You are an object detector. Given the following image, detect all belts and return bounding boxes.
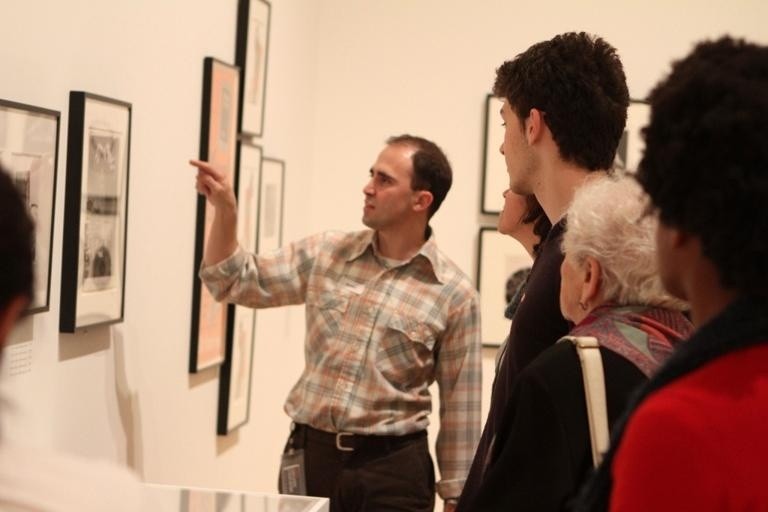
[294,422,394,454]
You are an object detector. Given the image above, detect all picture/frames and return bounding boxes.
[480,93,652,216]
[476,226,534,348]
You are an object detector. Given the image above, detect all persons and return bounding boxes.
[187,136,483,510]
[1,164,144,487]
[490,33,633,479]
[455,169,697,512]
[610,33,768,511]
[496,178,552,333]
